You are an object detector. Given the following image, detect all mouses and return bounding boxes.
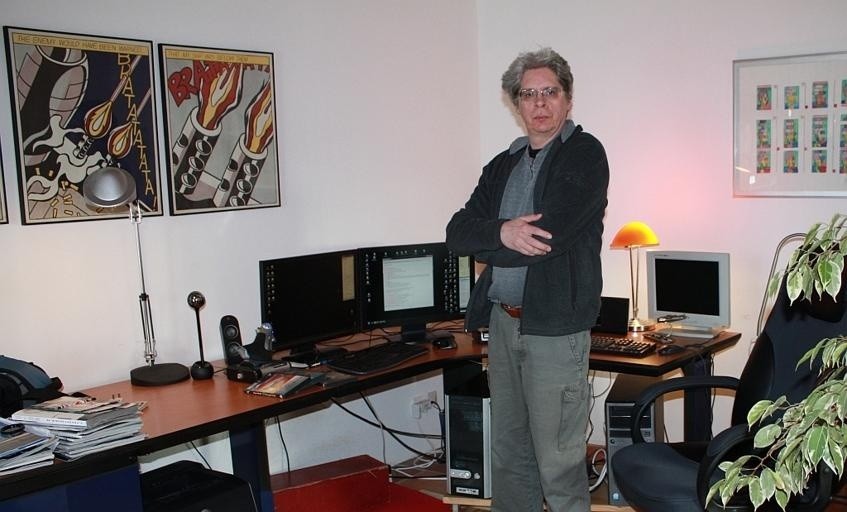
[658,344,682,356]
[428,329,457,350]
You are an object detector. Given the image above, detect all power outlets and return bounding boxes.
[412,394,429,418]
[425,390,439,412]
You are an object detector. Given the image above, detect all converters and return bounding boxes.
[410,401,420,419]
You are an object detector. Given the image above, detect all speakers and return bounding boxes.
[221,315,244,366]
[591,296,630,335]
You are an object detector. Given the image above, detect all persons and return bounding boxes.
[445,46,611,512]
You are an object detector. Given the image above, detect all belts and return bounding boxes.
[498,303,526,321]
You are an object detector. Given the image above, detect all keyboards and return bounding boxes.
[591,336,656,358]
[329,341,429,375]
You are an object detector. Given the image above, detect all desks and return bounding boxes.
[209,322,742,512]
[1,366,332,512]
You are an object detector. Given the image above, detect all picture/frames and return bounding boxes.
[155,43,282,216]
[732,50,847,199]
[4,27,164,227]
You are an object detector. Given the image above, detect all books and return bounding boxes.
[244,369,356,398]
[11,395,150,462]
[0,416,61,477]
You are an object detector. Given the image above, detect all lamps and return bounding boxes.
[608,224,660,332]
[83,167,189,387]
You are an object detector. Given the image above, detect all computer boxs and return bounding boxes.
[606,375,664,506]
[442,381,490,498]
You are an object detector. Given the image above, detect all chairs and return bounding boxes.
[611,242,846,511]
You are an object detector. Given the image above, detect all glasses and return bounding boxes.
[519,86,567,103]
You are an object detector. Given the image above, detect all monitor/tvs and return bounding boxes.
[645,251,731,339]
[358,242,460,344]
[258,249,358,369]
